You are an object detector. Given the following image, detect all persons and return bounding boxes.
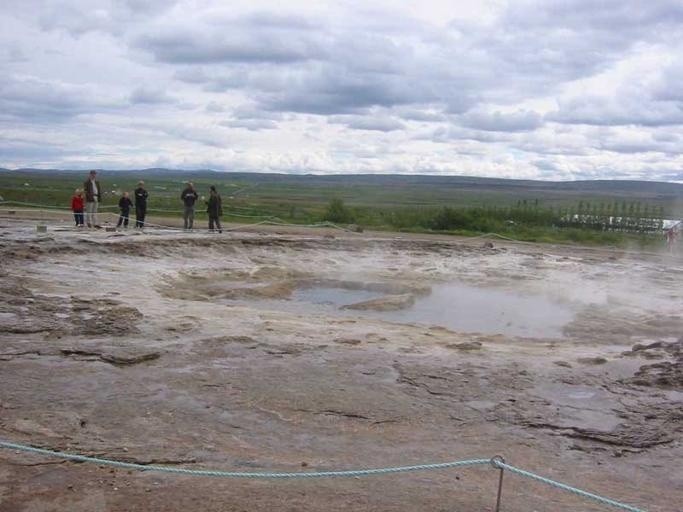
[115,191,133,230]
[132,180,148,230]
[179,182,198,232]
[82,170,100,228]
[200,185,222,233]
[69,188,85,227]
